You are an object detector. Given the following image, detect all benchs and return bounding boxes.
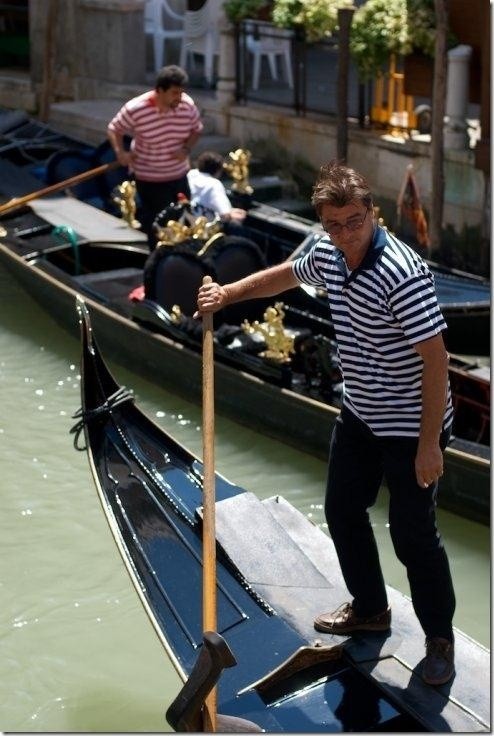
[46,135,144,220]
[144,235,312,374]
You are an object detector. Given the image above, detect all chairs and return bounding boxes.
[179,0,220,83]
[245,19,294,91]
[144,0,195,74]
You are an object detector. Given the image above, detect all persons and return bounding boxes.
[187,152,249,224]
[191,160,460,689]
[105,63,206,250]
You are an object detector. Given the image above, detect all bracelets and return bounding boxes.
[182,146,191,153]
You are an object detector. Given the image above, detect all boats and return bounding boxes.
[69,293,492,733]
[1,106,491,525]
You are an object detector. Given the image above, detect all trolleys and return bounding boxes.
[371,47,432,134]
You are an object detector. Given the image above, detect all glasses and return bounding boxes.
[321,209,367,235]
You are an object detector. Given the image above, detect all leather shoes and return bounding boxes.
[314,601,391,633]
[423,631,454,685]
